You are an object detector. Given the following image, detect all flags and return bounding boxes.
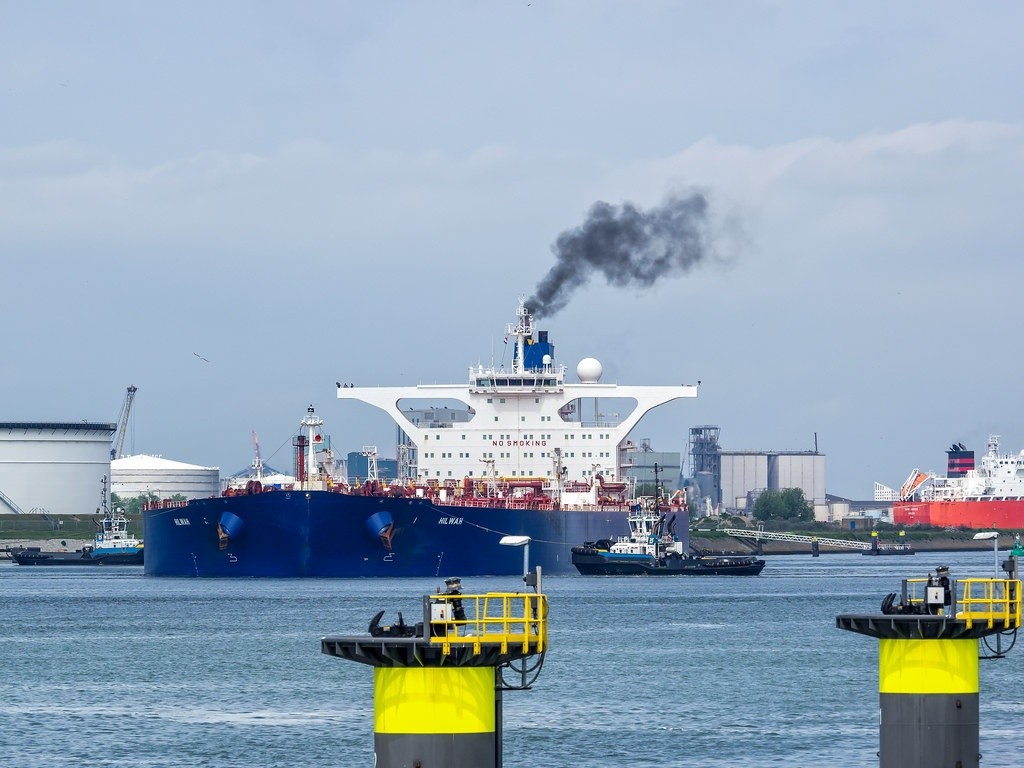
[504,335,508,344]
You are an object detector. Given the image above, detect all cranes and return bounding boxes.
[110,383,139,460]
[723,525,912,553]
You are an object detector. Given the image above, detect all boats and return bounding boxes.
[140,291,703,582]
[892,434,1024,533]
[569,458,767,578]
[1009,532,1024,559]
[5,473,143,567]
[596,473,626,493]
